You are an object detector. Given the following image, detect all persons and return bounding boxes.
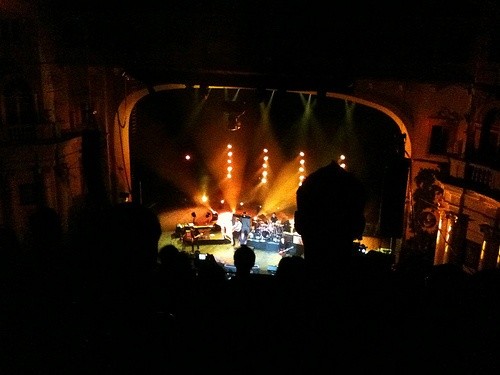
[393,249,427,279]
[20,202,63,252]
[267,257,312,299]
[254,161,418,375]
[1,194,231,374]
[157,206,305,281]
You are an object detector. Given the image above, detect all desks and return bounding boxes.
[247,238,285,252]
